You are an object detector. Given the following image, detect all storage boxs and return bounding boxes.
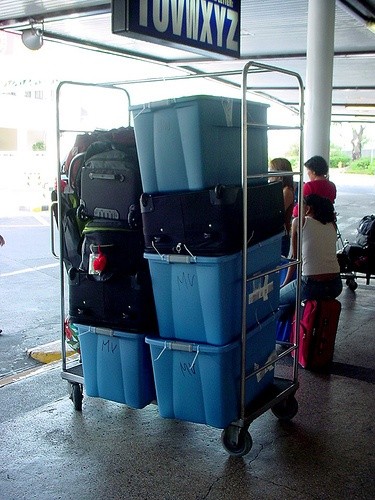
[144,307,285,428]
[143,229,287,345]
[72,323,154,409]
[128,94,270,193]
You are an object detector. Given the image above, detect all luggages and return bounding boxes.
[67,267,158,335]
[76,140,143,230]
[290,299,341,369]
[140,180,285,257]
[78,217,150,273]
[339,244,374,275]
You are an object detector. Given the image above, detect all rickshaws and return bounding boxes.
[52,60,305,458]
[335,224,375,290]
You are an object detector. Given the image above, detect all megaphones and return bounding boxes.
[21,28,44,50]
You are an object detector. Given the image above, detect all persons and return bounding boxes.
[279,194,342,307]
[270,158,294,257]
[292,155,336,217]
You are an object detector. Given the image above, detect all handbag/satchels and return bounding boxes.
[357,215,375,234]
[356,235,375,246]
[61,126,134,195]
[50,178,84,273]
